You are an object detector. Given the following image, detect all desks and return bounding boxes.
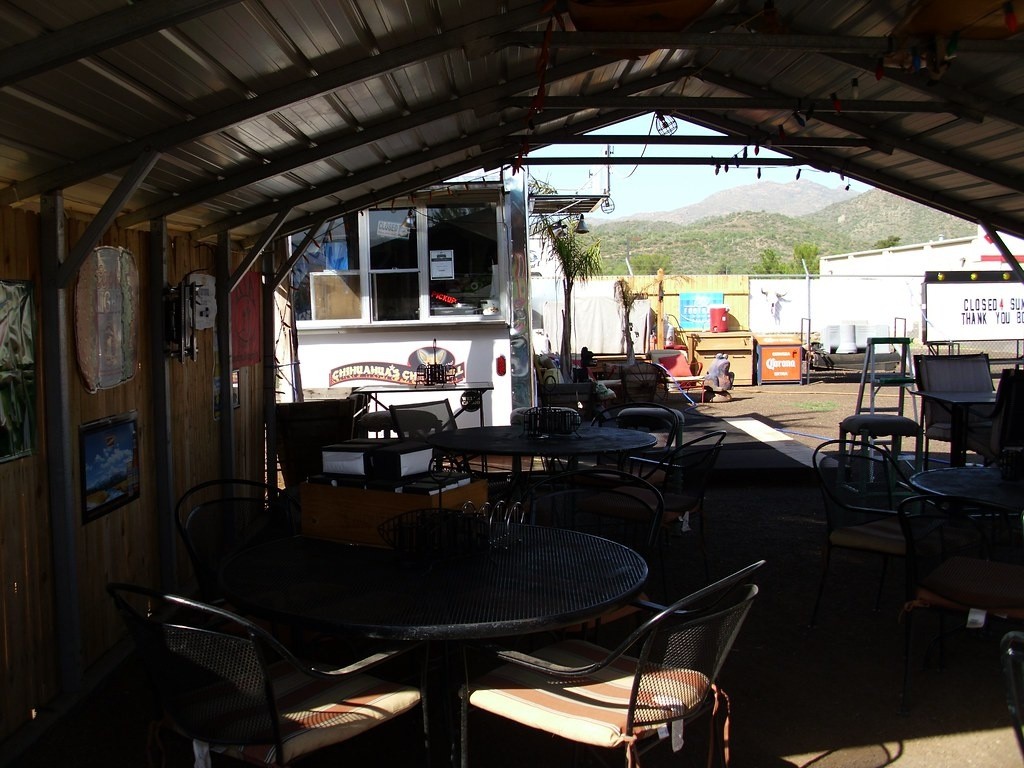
[351,381,494,475]
[217,519,649,768]
[909,464,1024,531]
[908,391,996,465]
[426,425,659,500]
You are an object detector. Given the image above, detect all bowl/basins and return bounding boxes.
[481,299,499,315]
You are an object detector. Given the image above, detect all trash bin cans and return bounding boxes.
[688,332,753,387]
[757,344,803,384]
[277,394,366,487]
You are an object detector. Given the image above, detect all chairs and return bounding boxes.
[177,478,361,672]
[646,349,705,403]
[456,559,766,768]
[811,351,1024,757]
[105,580,434,767]
[387,401,728,647]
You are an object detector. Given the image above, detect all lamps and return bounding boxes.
[575,213,589,234]
[164,281,199,362]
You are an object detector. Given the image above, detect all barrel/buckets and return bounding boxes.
[709,304,729,332]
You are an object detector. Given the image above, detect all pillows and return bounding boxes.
[659,354,693,377]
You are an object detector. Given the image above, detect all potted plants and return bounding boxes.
[525,179,607,416]
[602,274,695,406]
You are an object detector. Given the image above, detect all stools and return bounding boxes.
[839,417,923,512]
